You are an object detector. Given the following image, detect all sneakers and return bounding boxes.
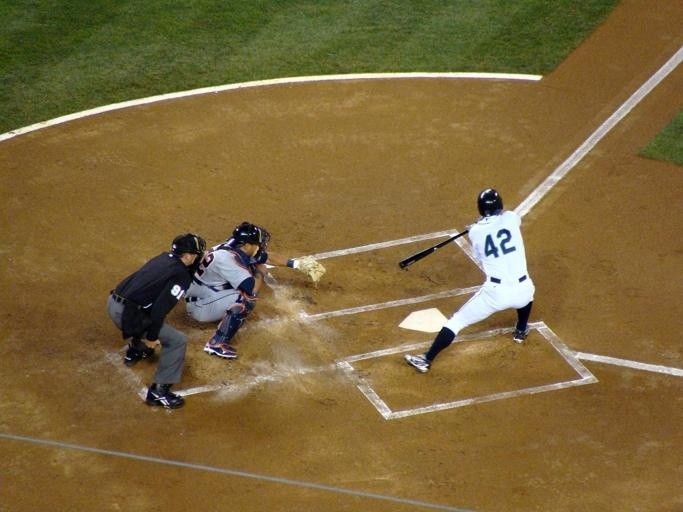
[122,343,157,367]
[146,387,186,409]
[404,353,432,374]
[513,324,530,344]
[204,337,239,361]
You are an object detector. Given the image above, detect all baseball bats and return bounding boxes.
[399,229,469,268]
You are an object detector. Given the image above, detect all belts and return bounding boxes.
[491,275,527,284]
[183,297,198,302]
[108,290,153,312]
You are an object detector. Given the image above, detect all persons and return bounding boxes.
[404,188,535,373]
[107,234,208,409]
[185,222,304,359]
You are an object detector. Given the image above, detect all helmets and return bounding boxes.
[171,233,206,255]
[232,221,270,247]
[476,187,504,218]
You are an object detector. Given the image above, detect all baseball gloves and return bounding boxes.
[293,257,326,281]
